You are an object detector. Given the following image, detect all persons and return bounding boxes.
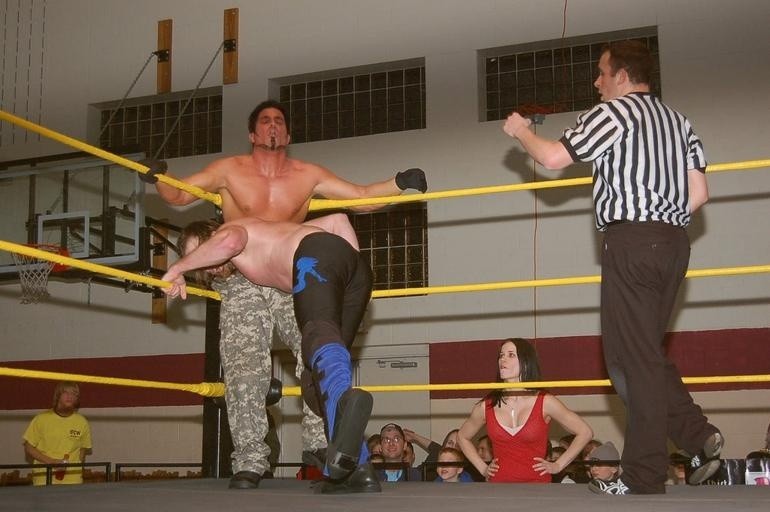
[503,43,724,493]
[457,338,594,483]
[366,423,620,483]
[159,213,381,495]
[24,383,92,485]
[664,449,692,486]
[131,100,428,489]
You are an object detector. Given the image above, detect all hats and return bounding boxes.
[589,442,619,461]
[381,424,403,437]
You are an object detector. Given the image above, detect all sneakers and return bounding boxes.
[589,476,637,496]
[686,433,723,485]
[228,471,260,489]
[310,389,381,480]
[314,463,382,494]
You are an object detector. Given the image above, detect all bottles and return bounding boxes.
[55,453,69,480]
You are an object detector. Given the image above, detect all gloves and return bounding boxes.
[395,168,427,194]
[129,159,167,183]
